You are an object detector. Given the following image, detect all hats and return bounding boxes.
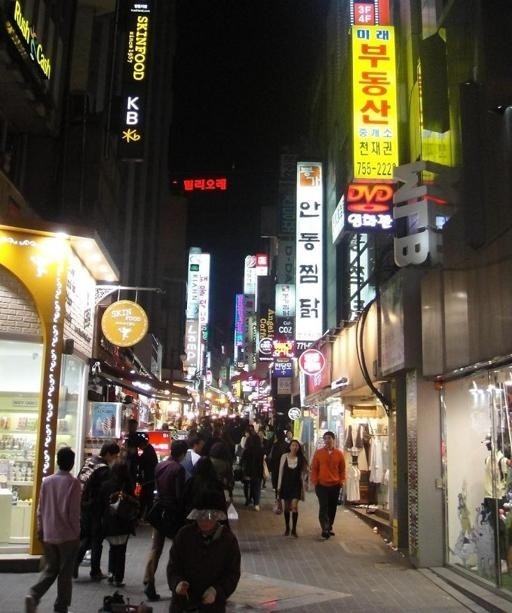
[186,488,228,521]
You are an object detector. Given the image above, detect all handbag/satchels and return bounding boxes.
[147,501,177,538]
[109,479,141,521]
[272,489,283,514]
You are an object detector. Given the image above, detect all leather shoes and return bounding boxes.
[321,531,329,537]
[329,529,334,535]
[144,582,160,600]
[92,571,107,578]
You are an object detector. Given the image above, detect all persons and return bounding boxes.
[166,486,242,611]
[23,446,83,612]
[142,436,235,602]
[78,444,140,586]
[187,412,293,510]
[310,431,348,540]
[275,438,309,539]
[153,412,189,430]
[481,432,509,575]
[119,418,159,522]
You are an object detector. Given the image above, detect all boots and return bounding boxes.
[284,511,289,535]
[291,511,298,537]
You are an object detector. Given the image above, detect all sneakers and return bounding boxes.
[24,594,37,612]
[254,504,260,511]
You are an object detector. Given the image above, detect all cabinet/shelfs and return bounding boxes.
[0,391,78,544]
